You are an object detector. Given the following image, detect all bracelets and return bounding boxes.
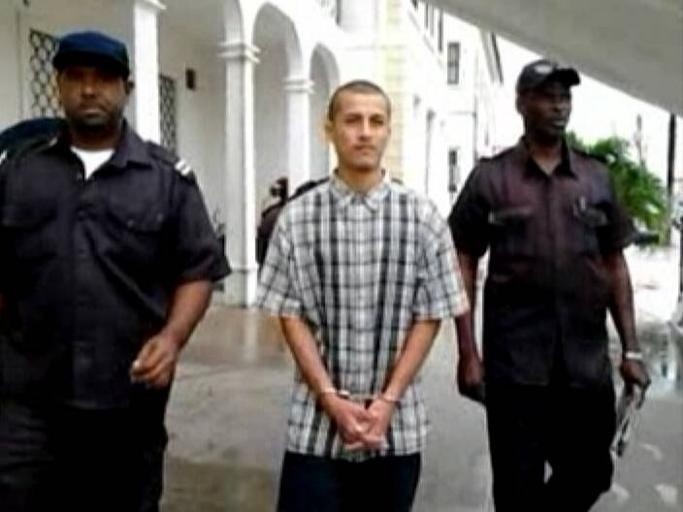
[621,350,643,362]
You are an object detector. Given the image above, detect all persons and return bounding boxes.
[444,58,654,511]
[0,30,233,511]
[247,78,473,512]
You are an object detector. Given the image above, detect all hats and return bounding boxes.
[51,31,130,81]
[517,59,580,94]
